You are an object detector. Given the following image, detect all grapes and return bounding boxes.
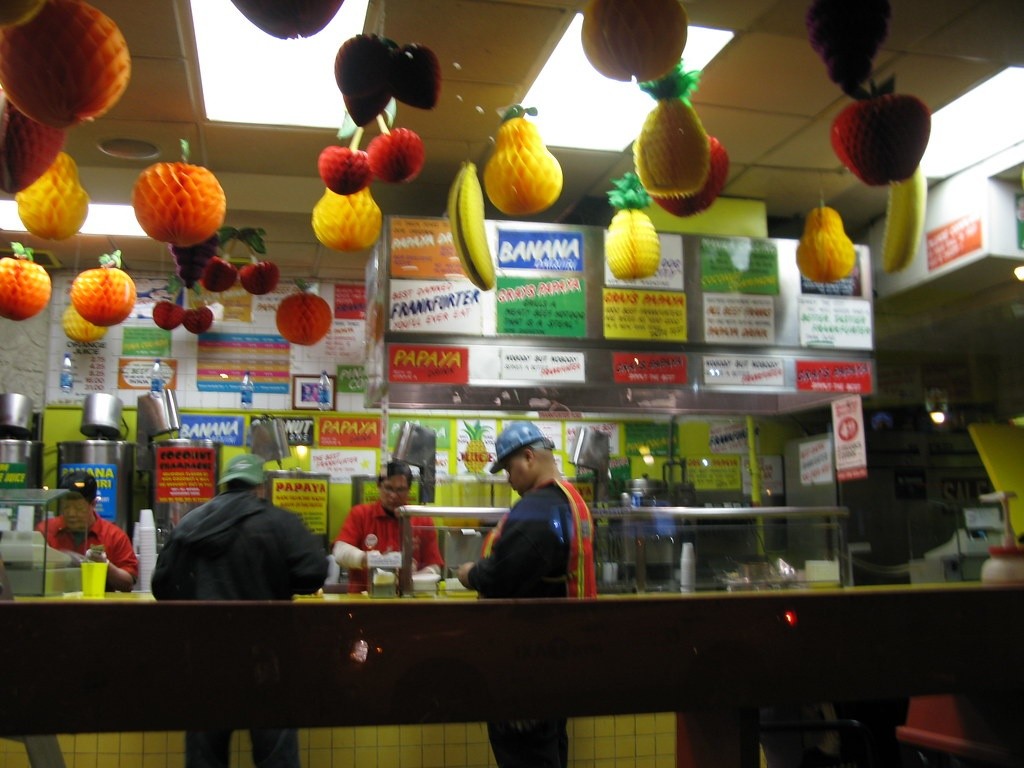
[806,0,891,92]
[169,232,219,290]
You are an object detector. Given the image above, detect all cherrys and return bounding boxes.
[318,110,424,194]
[151,278,213,334]
[198,233,279,295]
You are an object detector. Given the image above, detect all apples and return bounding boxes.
[828,78,931,183]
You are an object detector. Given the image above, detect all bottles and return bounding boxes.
[318,370,332,411]
[981,545,1024,584]
[60,352,74,403]
[150,359,162,392]
[241,372,254,409]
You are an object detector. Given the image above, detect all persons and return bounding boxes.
[150,453,329,767]
[459,421,596,768]
[37,471,138,594]
[331,462,446,594]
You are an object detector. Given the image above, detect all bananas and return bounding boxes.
[448,161,495,292]
[883,169,926,271]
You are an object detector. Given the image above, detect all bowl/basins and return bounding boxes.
[412,573,441,598]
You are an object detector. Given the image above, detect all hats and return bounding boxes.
[217,455,268,485]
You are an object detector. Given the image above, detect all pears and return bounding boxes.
[483,109,562,215]
[796,191,855,283]
[312,181,382,253]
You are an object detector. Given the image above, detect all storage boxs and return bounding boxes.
[0,489,88,596]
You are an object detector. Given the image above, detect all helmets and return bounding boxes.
[489,421,546,475]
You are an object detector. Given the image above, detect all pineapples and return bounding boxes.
[632,55,712,198]
[604,171,661,279]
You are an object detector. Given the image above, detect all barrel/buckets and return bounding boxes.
[0,389,331,550]
[395,421,437,469]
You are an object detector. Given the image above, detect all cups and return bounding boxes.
[680,542,695,593]
[132,509,158,593]
[601,561,618,583]
[81,562,108,599]
